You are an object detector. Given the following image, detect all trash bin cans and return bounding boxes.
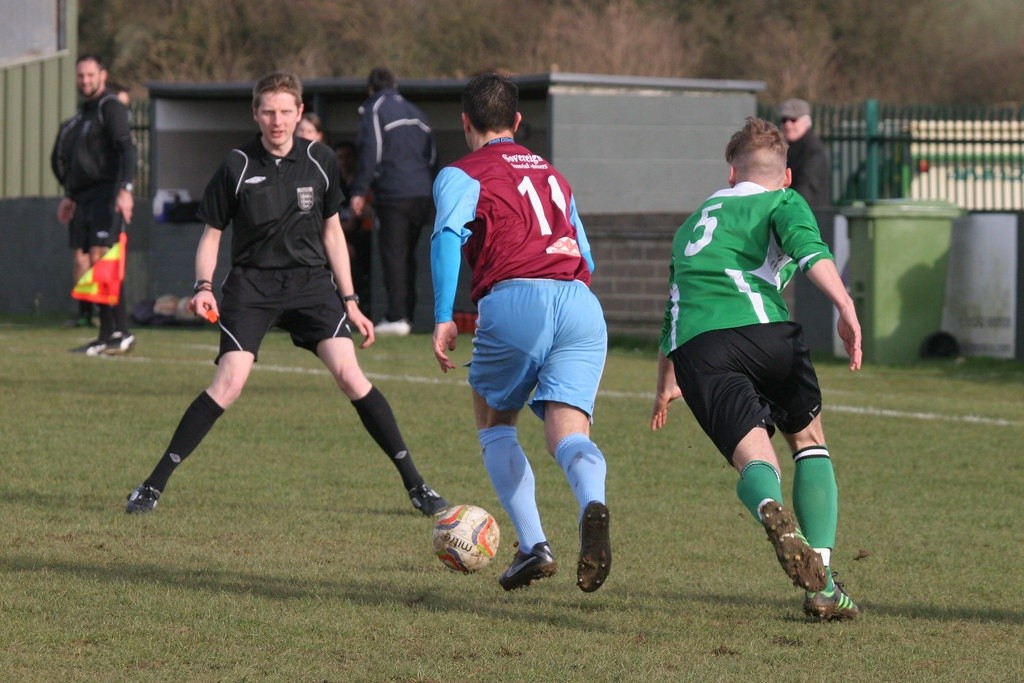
[840,198,960,367]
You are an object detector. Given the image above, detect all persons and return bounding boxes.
[51,55,139,358]
[432,72,613,592]
[122,65,448,517]
[652,99,862,621]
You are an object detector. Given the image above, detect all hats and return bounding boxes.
[775,98,811,121]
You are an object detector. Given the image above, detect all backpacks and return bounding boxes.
[51,110,109,195]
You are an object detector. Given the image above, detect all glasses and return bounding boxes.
[780,118,797,123]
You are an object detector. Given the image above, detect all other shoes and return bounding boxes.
[73,332,138,356]
[372,318,412,335]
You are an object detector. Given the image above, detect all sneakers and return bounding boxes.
[758,500,827,593]
[408,480,448,518]
[123,484,162,515]
[804,572,859,619]
[499,541,558,591]
[577,500,612,593]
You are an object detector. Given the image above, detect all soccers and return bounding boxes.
[431,504,502,573]
[153,293,199,320]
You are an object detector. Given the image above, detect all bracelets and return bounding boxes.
[195,287,213,292]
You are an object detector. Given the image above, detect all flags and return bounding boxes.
[69,232,128,305]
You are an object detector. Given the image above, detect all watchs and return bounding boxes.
[342,294,360,305]
[123,182,133,192]
[194,280,213,289]
